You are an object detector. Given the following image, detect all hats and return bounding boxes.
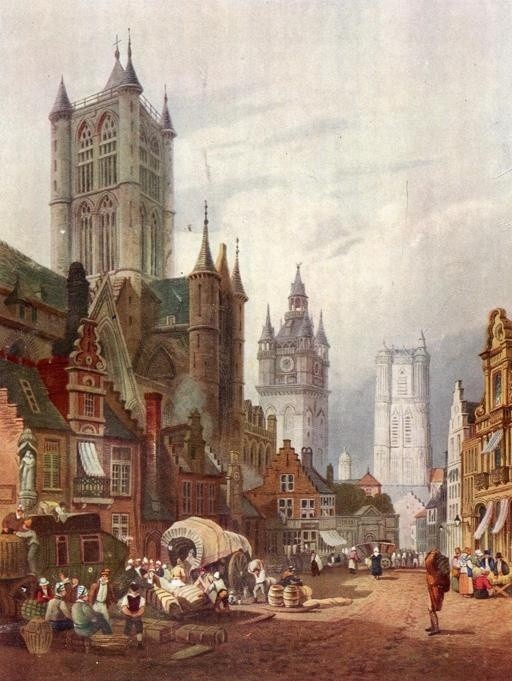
[55,582,64,593]
[483,550,489,554]
[100,568,111,575]
[76,585,88,599]
[495,553,504,558]
[252,568,259,572]
[39,577,49,586]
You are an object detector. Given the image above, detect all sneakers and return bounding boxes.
[425,627,440,636]
